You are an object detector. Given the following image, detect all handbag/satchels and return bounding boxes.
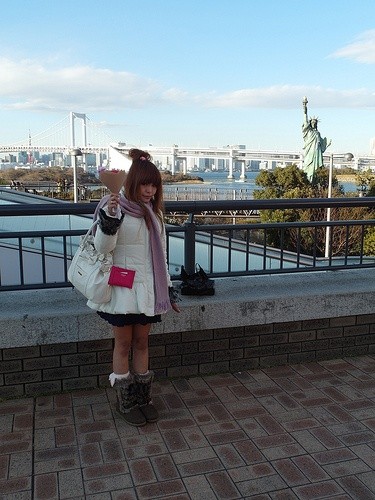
[66,218,112,304]
[180,263,216,296]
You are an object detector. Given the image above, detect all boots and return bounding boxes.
[133,370,159,423]
[110,373,147,427]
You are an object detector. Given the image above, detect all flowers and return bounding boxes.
[95,165,127,216]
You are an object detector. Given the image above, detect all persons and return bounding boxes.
[11,180,24,186]
[86,148,183,427]
[57,179,87,200]
[300,96,331,183]
